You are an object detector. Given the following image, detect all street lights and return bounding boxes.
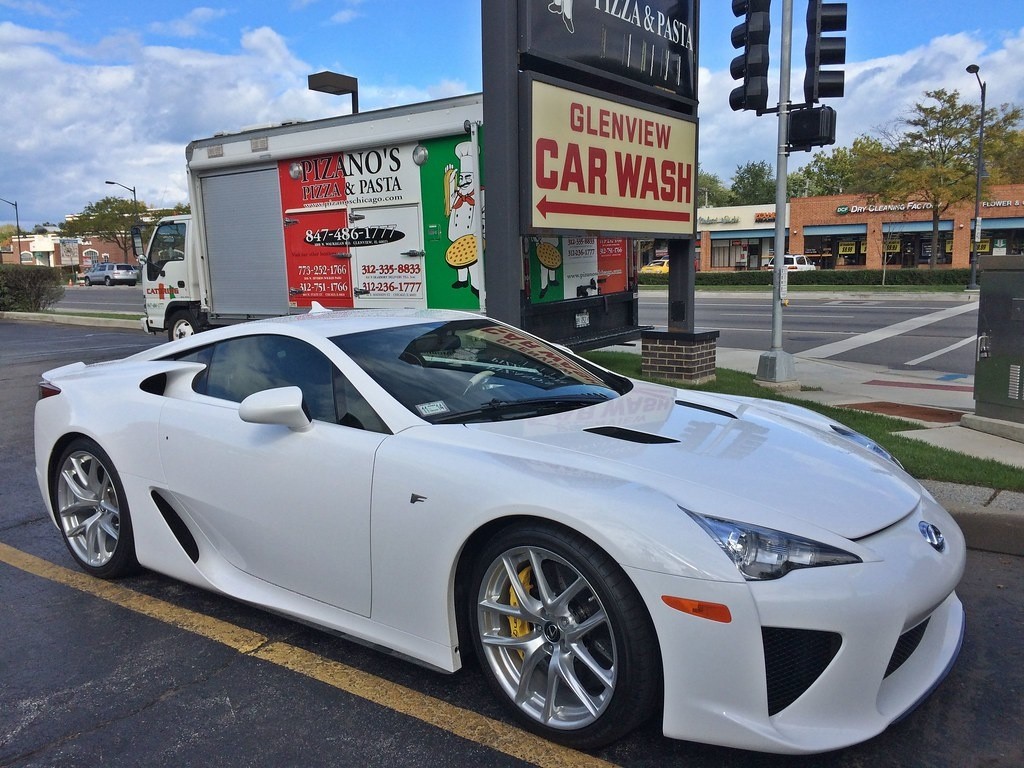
[107,179,140,226]
[963,63,989,290]
[0,198,22,264]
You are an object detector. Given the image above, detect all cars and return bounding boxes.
[84,262,138,286]
[767,254,816,271]
[769,254,769,255]
[641,255,669,274]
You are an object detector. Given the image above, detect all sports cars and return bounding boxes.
[33,303,969,759]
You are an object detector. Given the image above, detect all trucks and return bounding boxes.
[139,89,657,357]
